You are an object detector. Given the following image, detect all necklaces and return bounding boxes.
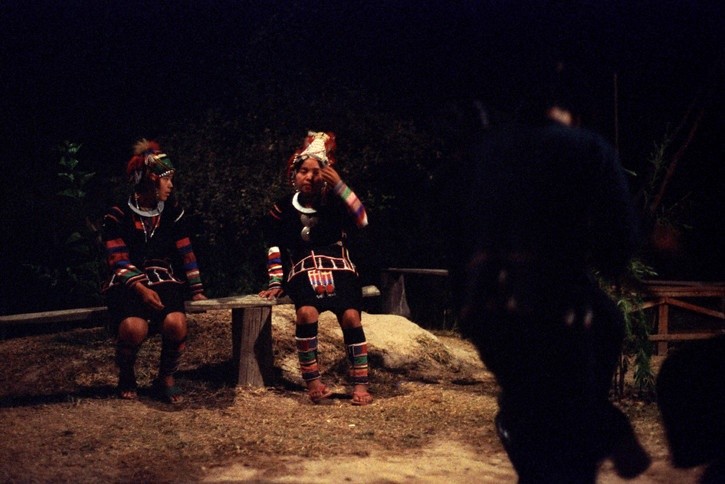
[293,191,330,243]
[126,192,166,217]
[135,192,163,246]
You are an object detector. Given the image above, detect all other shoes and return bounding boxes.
[118,381,139,399]
[161,384,184,403]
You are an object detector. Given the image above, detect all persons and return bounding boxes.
[433,68,659,484]
[258,130,378,407]
[100,140,209,406]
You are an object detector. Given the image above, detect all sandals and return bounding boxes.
[308,384,332,403]
[350,391,374,405]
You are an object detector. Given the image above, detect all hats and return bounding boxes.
[286,129,338,199]
[126,137,176,203]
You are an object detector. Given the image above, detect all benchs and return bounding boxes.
[132,280,382,389]
